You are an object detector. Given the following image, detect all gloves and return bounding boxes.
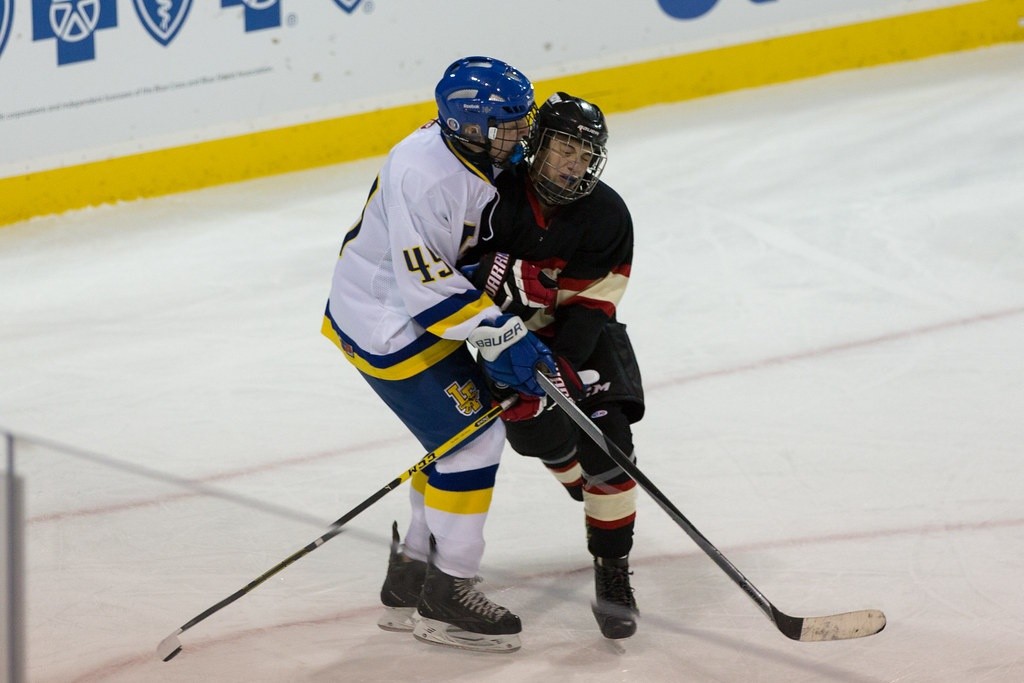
[490,353,585,422]
[466,314,557,397]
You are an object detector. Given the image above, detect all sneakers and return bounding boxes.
[377,521,429,629]
[412,534,523,653]
[592,555,639,652]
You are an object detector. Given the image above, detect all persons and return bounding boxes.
[321,55,559,653]
[477,92,644,652]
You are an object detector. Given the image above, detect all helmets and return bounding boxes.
[528,92,607,206]
[434,56,538,168]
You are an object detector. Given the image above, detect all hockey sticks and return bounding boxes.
[155,394,520,665]
[536,368,886,643]
